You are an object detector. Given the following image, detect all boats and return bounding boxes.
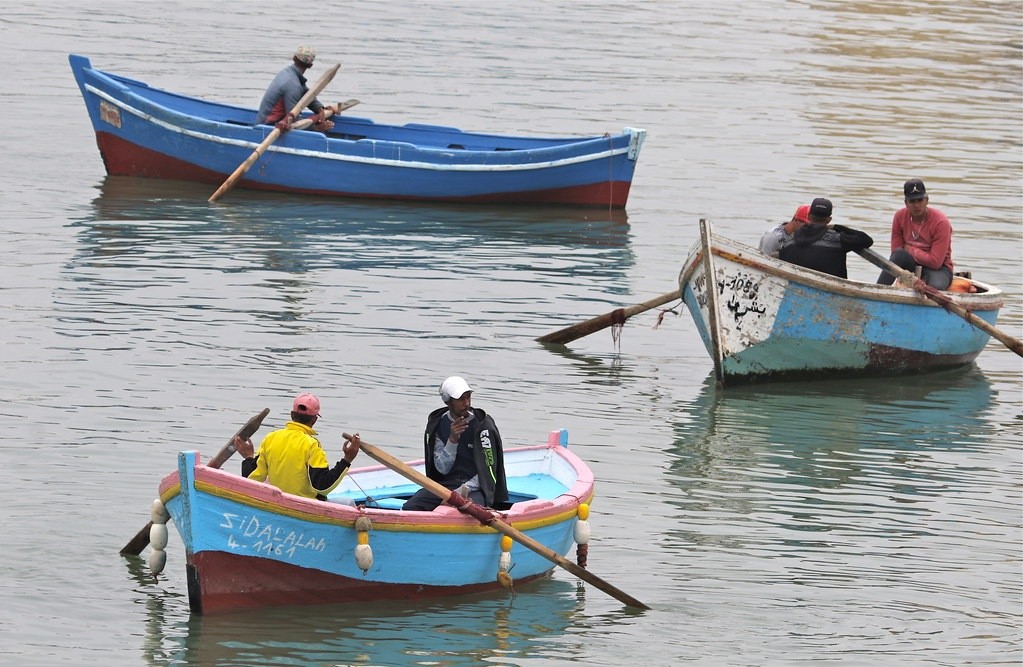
[161,429,598,621]
[674,217,1005,388]
[67,54,644,216]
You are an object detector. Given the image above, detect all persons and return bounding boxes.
[781,198,873,279]
[759,204,812,258]
[401,376,507,510]
[255,46,334,132]
[877,179,953,289]
[233,393,360,501]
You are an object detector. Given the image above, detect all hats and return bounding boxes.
[293,392,322,419]
[295,47,316,64]
[441,376,475,400]
[810,198,833,217]
[904,178,926,199]
[794,205,811,224]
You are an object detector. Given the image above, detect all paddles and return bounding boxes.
[534,290,679,344]
[119,408,270,554]
[338,430,649,609]
[208,62,360,202]
[854,247,1023,358]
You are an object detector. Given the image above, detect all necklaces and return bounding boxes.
[910,216,925,240]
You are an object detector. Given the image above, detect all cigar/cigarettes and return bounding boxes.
[355,435,360,439]
[467,410,473,415]
